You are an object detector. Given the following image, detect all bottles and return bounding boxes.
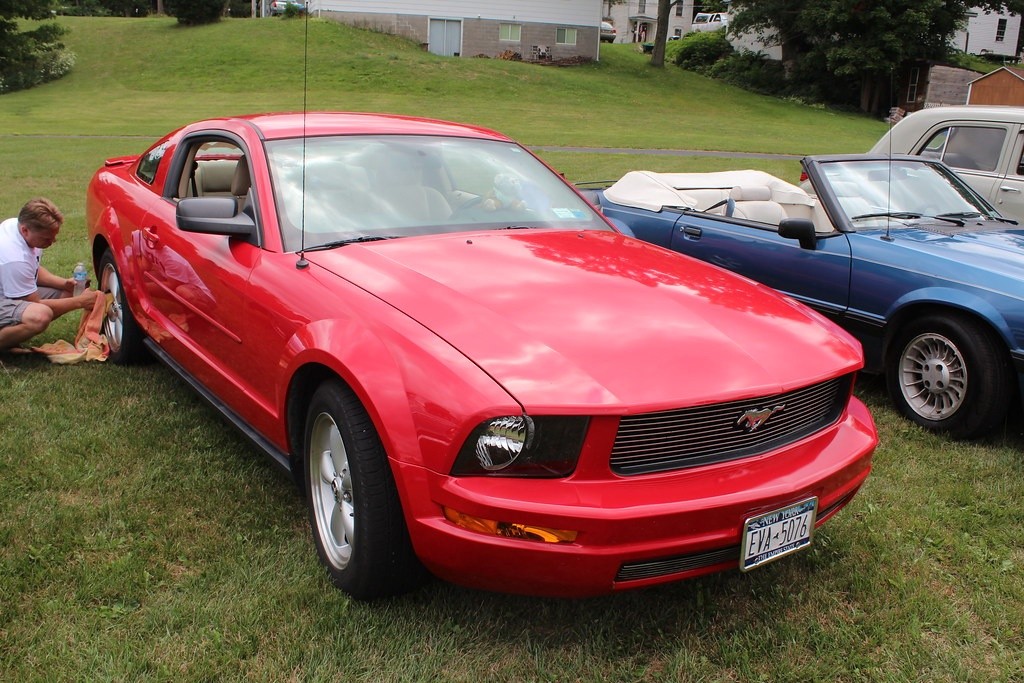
[72,262,88,297]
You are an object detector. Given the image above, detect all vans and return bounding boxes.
[691,12,728,34]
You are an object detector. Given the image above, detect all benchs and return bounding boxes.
[188,159,240,197]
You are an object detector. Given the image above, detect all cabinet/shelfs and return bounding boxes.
[532,45,552,62]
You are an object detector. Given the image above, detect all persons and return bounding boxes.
[631,25,647,44]
[0,197,112,354]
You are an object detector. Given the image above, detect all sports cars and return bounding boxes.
[571,152,1024,440]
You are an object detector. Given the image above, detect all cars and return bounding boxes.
[800,105,1024,231]
[85,111,878,603]
[600,22,617,43]
[269,0,302,17]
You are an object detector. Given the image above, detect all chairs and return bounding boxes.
[231,154,251,216]
[722,184,789,225]
[811,182,878,233]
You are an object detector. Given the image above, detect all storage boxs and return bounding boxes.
[889,107,905,122]
